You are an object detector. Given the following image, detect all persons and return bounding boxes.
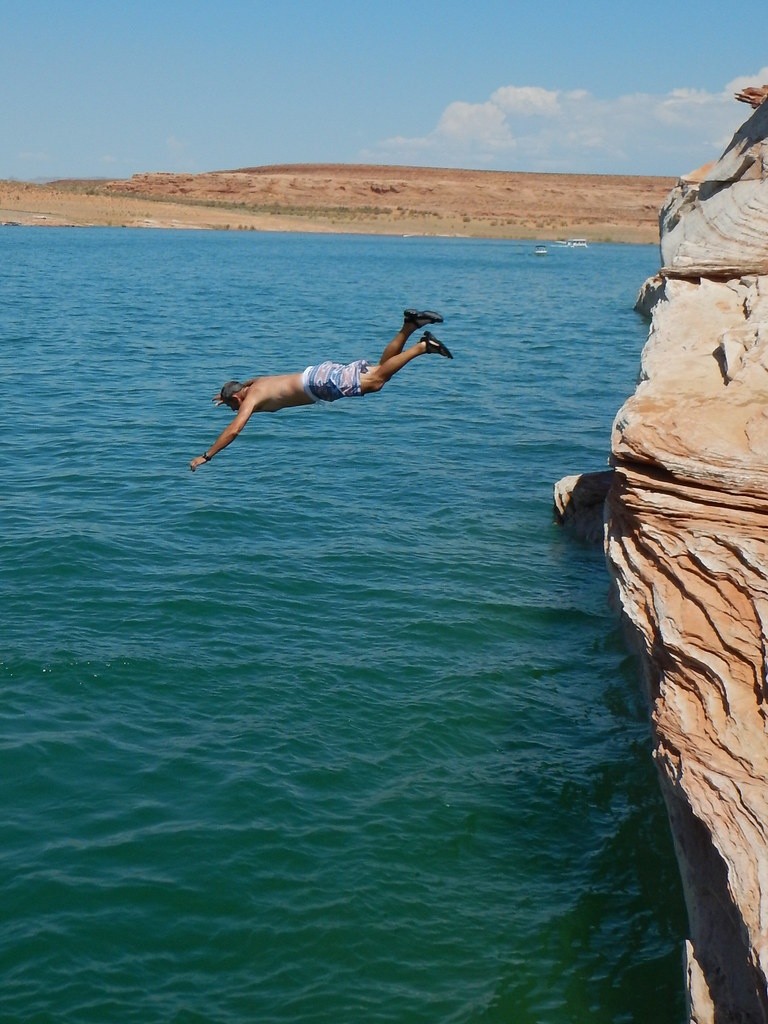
[190,309,454,473]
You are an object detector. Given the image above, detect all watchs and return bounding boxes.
[202,451,211,462]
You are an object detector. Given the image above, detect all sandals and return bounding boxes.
[404,309,444,328]
[420,331,454,359]
